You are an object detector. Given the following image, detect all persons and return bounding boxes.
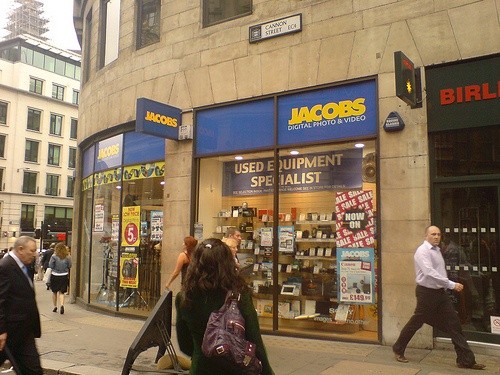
[392,225,486,370]
[0,235,72,314]
[438,232,491,332]
[0,236,44,375]
[165,227,276,375]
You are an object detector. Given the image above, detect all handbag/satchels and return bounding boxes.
[43,268,52,283]
[201,288,262,375]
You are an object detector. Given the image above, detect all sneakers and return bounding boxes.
[395,352,408,363]
[458,363,484,369]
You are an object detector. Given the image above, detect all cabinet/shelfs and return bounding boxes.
[294,220,336,260]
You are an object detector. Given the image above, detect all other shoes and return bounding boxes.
[53,307,57,312]
[61,306,64,314]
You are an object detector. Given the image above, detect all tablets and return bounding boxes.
[281,285,295,294]
